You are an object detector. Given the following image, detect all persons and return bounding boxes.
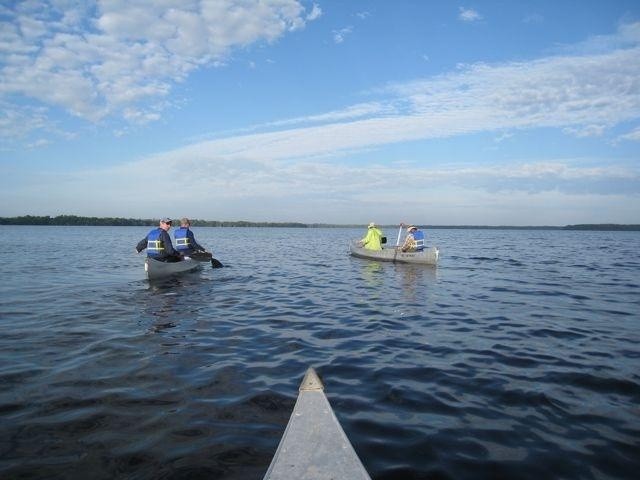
[134,216,191,263]
[355,222,383,250]
[401,224,424,252]
[173,217,207,255]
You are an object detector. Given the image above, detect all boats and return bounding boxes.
[348,239,440,265]
[143,252,203,278]
[261,364,372,480]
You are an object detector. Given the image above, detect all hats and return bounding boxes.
[180,217,190,224]
[407,224,417,233]
[160,217,173,223]
[368,223,377,228]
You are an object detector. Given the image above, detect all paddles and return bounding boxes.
[141,249,212,263]
[202,250,222,266]
